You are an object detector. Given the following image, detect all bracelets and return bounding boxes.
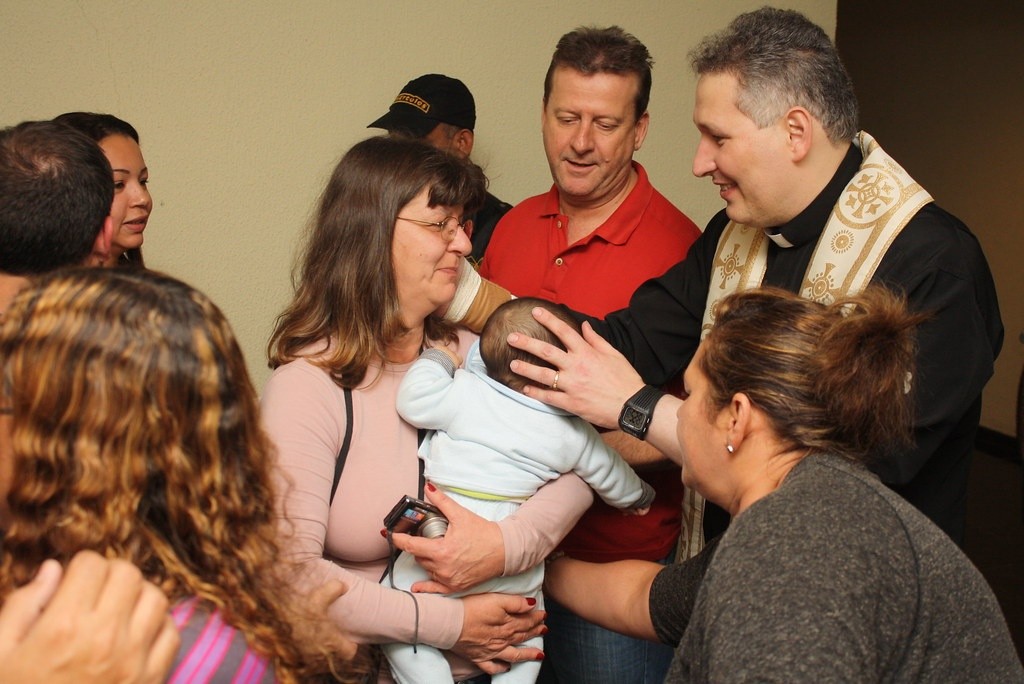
[542,550,564,594]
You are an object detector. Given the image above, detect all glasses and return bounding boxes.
[395,213,474,243]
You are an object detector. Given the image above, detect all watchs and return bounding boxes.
[618,385,668,441]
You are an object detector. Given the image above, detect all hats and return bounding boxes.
[367,73,475,139]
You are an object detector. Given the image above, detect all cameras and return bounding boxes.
[384,495,449,539]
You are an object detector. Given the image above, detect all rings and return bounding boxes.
[553,371,560,391]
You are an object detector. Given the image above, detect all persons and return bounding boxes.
[542,286,1024,684]
[257,136,596,684]
[379,296,656,684]
[477,25,703,684]
[366,73,515,270]
[0,111,375,684]
[428,7,1003,567]
